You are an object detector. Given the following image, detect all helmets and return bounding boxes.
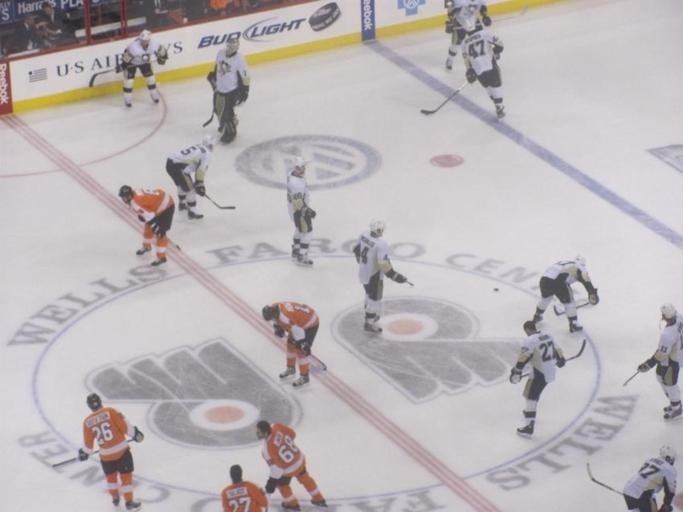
[202,135,216,152]
[660,445,675,465]
[87,394,101,409]
[225,39,240,55]
[140,29,151,41]
[370,219,385,237]
[263,305,276,320]
[661,303,676,319]
[119,185,133,204]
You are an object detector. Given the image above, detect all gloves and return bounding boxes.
[79,449,88,461]
[265,479,276,493]
[639,363,650,373]
[196,186,205,196]
[275,329,284,337]
[510,368,522,384]
[158,58,166,64]
[133,427,144,442]
[395,274,407,283]
[306,208,315,218]
[445,22,454,33]
[466,68,476,83]
[588,289,598,305]
[556,358,565,368]
[116,61,128,72]
[296,339,310,356]
[482,16,491,26]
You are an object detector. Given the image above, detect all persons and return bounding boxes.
[207,37,250,143]
[460,19,505,118]
[445,0,492,70]
[263,301,319,387]
[353,226,407,332]
[222,465,268,512]
[119,185,175,266]
[256,420,325,509]
[166,135,216,219]
[623,448,678,512]
[509,321,566,434]
[7,0,258,54]
[78,394,144,510]
[116,30,168,107]
[637,305,683,419]
[532,254,599,333]
[286,157,316,264]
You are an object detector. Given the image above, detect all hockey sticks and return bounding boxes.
[586,464,623,499]
[422,80,467,114]
[553,303,590,316]
[520,341,586,377]
[203,98,216,127]
[280,332,327,373]
[89,70,117,88]
[204,194,234,209]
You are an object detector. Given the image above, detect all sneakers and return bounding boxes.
[533,315,543,323]
[136,248,151,254]
[113,499,141,509]
[279,368,295,378]
[498,111,505,118]
[151,257,166,265]
[179,203,203,219]
[664,400,682,418]
[293,375,309,387]
[292,249,313,264]
[446,58,452,70]
[517,425,533,434]
[365,316,382,332]
[570,325,582,333]
